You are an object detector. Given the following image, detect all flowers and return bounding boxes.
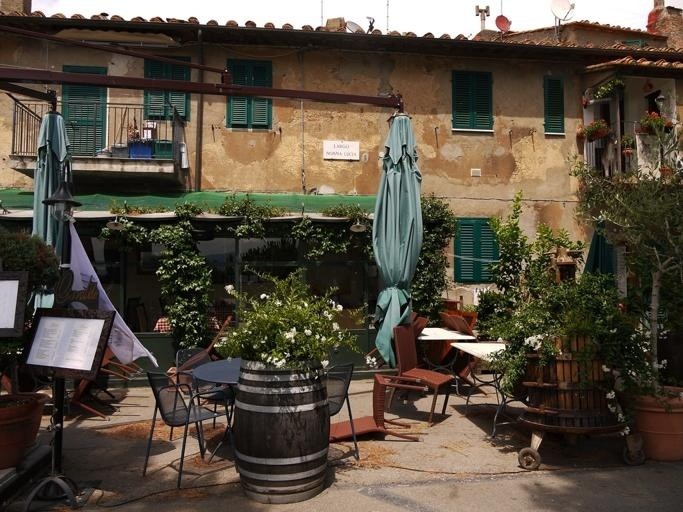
[595,81,613,101]
[583,120,613,142]
[213,262,377,375]
[639,110,673,128]
[479,273,669,441]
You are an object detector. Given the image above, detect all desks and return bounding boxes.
[191,357,243,472]
[450,340,508,439]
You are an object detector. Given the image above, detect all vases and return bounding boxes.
[592,128,607,139]
[635,125,650,133]
[664,127,673,133]
[0,392,36,470]
[228,355,333,503]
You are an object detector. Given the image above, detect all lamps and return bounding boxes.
[105,217,123,232]
[349,217,368,233]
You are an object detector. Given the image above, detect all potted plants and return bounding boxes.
[0,228,63,445]
[565,153,682,464]
[621,135,633,158]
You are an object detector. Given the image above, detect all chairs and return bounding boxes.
[140,346,235,491]
[325,308,481,461]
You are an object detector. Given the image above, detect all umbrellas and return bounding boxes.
[0,24,424,369]
[0,80,73,316]
